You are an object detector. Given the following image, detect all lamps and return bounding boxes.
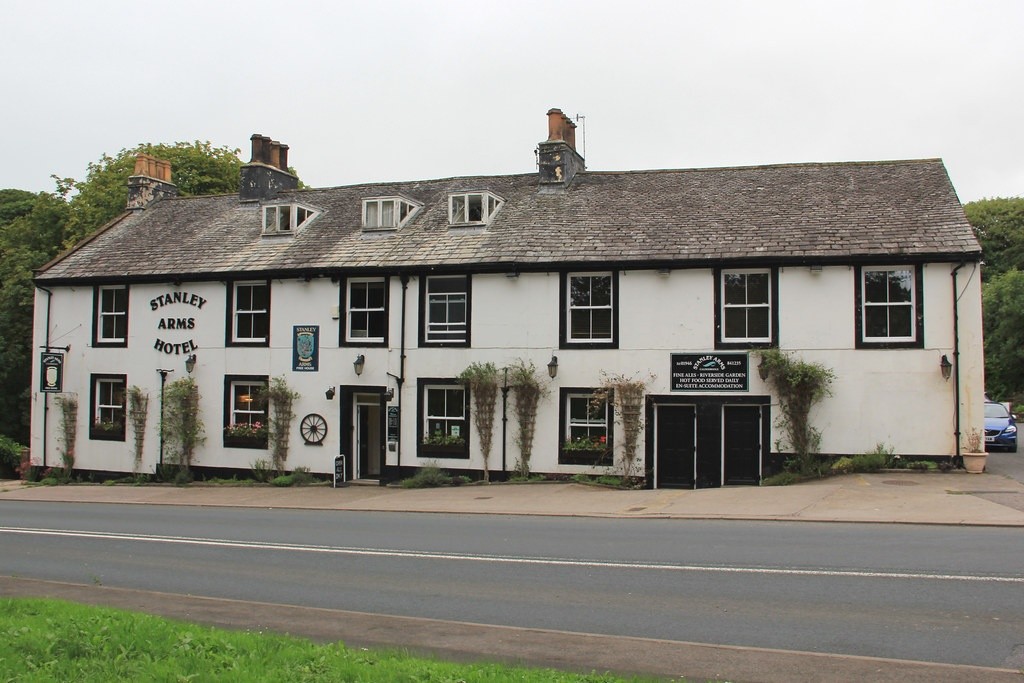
[353,354,365,378]
[384,387,394,401]
[757,355,770,382]
[547,356,558,381]
[657,268,671,276]
[810,266,822,272]
[293,276,310,284]
[325,386,335,399]
[505,272,520,280]
[167,282,183,287]
[185,353,196,374]
[939,355,952,382]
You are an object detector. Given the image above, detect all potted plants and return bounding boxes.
[419,434,468,452]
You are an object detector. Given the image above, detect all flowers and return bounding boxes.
[563,435,607,453]
[224,422,267,439]
[90,417,122,432]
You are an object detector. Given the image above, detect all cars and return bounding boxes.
[983,402,1018,452]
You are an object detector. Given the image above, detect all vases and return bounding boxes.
[963,452,988,474]
[562,447,612,459]
[91,429,123,437]
[224,437,267,446]
